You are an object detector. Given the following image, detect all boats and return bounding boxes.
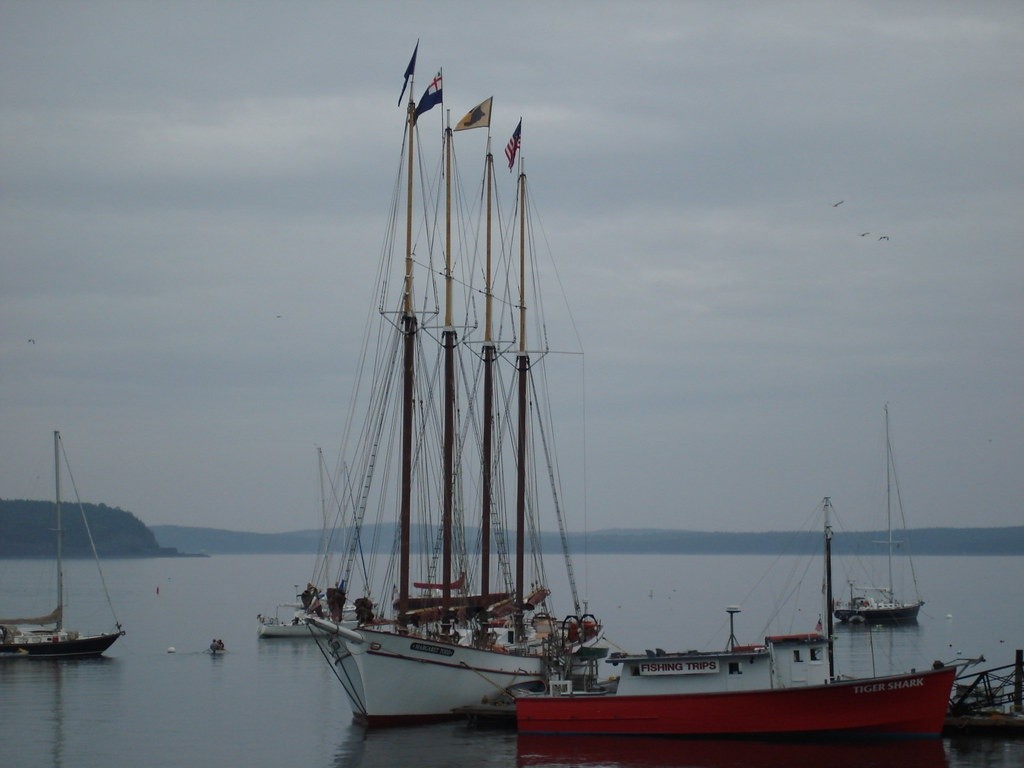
[515,733,952,768]
[513,497,959,739]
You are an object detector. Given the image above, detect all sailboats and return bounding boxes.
[256,446,378,640]
[833,404,924,627]
[1,429,127,661]
[302,37,606,724]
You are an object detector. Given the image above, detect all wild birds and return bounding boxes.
[879,236,890,241]
[829,201,844,207]
[856,232,870,237]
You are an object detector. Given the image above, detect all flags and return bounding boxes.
[453,98,491,132]
[397,45,416,106]
[413,72,442,127]
[504,122,521,175]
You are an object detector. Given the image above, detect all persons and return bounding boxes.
[210,638,224,650]
